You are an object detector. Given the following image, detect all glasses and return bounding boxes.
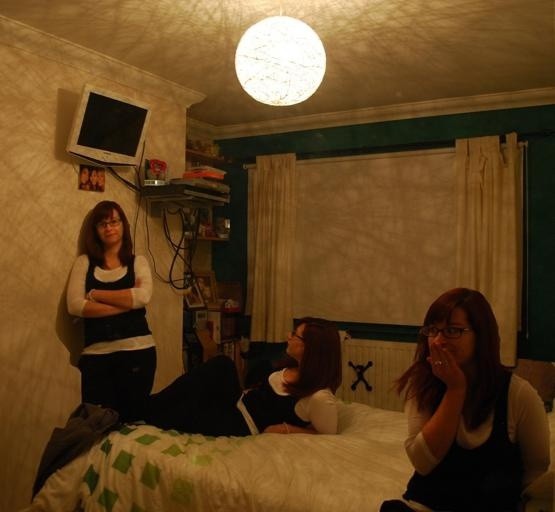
[420,325,470,338]
[292,331,303,341]
[98,218,121,230]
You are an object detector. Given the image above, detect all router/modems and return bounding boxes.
[143,179,165,187]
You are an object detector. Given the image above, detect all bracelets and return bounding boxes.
[281,421,290,433]
[88,288,95,303]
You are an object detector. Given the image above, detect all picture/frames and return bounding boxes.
[192,270,219,303]
[182,273,206,311]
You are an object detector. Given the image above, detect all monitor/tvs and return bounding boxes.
[65,83,151,169]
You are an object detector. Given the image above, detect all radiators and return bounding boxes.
[335,334,418,414]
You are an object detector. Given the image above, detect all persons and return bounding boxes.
[77,165,106,192]
[379,286,555,510]
[62,200,157,412]
[138,315,345,439]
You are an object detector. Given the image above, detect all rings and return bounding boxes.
[435,360,449,366]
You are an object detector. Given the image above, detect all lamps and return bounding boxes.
[234,4,328,107]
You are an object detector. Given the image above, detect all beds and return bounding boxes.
[2,391,553,512]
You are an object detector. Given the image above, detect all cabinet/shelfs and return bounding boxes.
[184,146,234,243]
